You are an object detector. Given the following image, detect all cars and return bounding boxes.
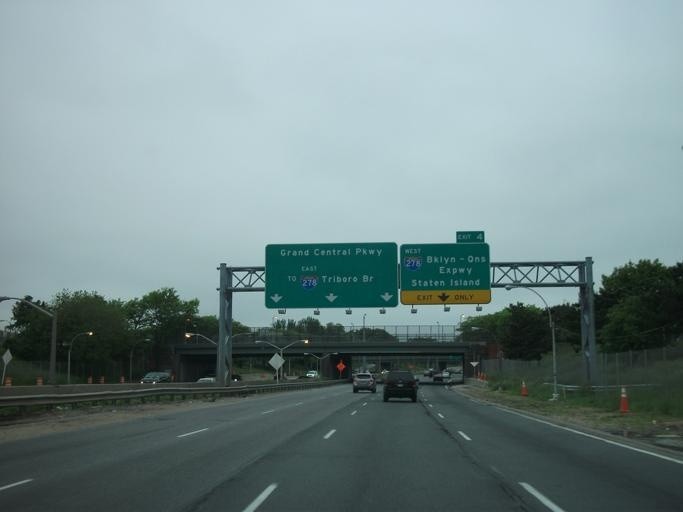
[197,378,215,383]
[351,368,451,402]
[306,371,318,378]
[231,374,242,381]
[139,372,171,383]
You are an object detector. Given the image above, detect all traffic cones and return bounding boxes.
[620,384,629,412]
[477,370,486,380]
[521,381,527,396]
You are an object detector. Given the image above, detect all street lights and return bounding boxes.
[304,353,338,371]
[67,332,93,384]
[0,297,57,384]
[505,285,557,394]
[185,333,216,345]
[129,339,151,382]
[255,340,308,380]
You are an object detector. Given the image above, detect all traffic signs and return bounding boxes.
[265,231,491,308]
